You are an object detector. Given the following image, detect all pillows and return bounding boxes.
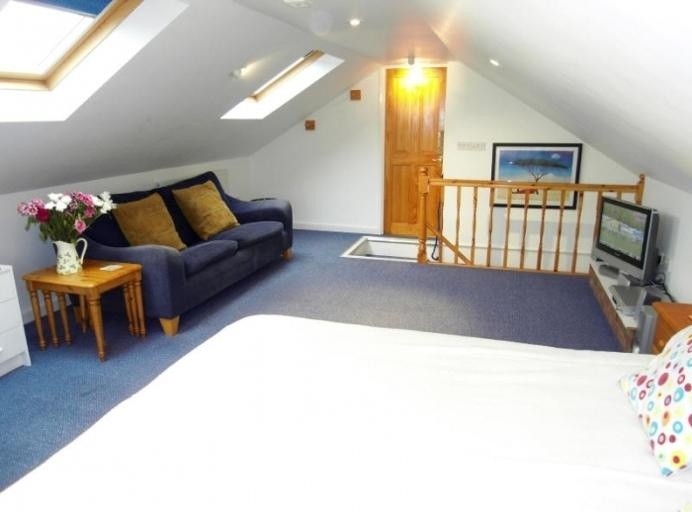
[170,180,241,242]
[619,324,692,476]
[109,192,187,253]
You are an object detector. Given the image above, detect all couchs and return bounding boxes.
[52,170,294,337]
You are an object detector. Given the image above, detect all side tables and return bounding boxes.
[22,257,145,362]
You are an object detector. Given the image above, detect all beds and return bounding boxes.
[1,315,692,512]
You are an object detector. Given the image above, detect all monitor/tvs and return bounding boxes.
[590,196,659,286]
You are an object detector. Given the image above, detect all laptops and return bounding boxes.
[609,285,672,317]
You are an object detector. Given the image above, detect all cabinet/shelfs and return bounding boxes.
[588,262,672,352]
[0,265,32,378]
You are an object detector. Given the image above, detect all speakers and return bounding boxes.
[633,288,661,321]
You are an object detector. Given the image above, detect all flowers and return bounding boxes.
[16,188,114,245]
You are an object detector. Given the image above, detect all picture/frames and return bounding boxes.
[488,142,582,210]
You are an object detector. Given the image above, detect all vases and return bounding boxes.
[51,236,88,275]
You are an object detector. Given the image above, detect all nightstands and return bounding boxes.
[647,302,692,354]
